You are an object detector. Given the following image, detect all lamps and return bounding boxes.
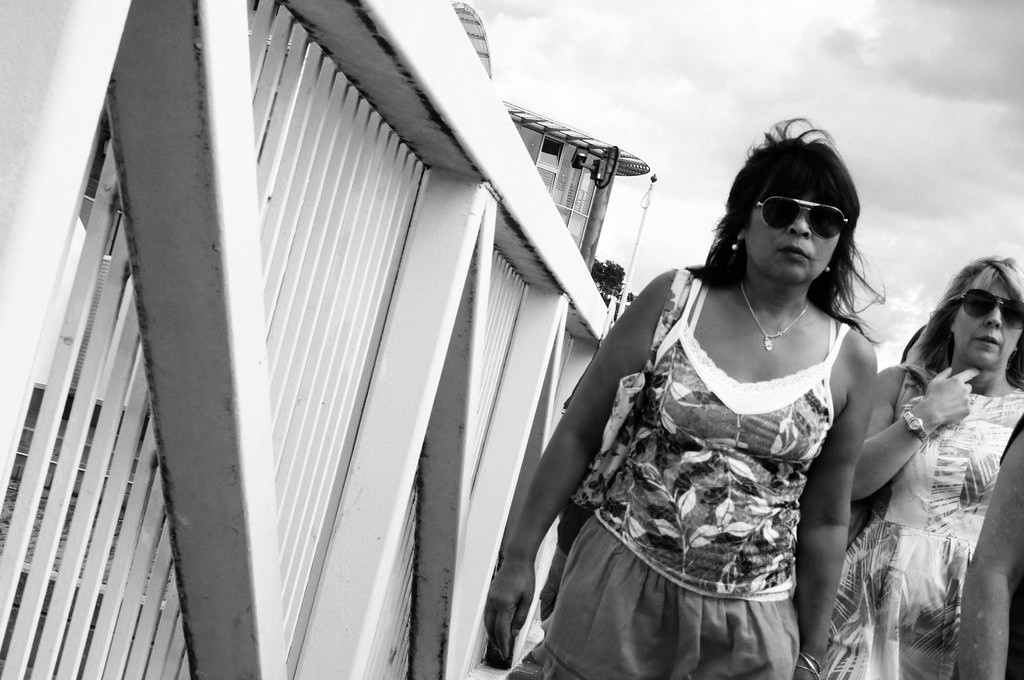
[570,145,621,188]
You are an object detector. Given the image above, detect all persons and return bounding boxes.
[484,119,877,680]
[958,413,1024,680]
[821,257,1024,680]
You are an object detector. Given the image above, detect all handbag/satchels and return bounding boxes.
[571,369,651,511]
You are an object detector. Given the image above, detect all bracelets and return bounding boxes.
[796,652,821,680]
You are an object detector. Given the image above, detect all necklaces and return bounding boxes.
[741,280,809,351]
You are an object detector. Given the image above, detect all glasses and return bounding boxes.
[958,288,1024,330]
[757,197,849,240]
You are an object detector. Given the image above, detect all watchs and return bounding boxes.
[903,411,929,443]
